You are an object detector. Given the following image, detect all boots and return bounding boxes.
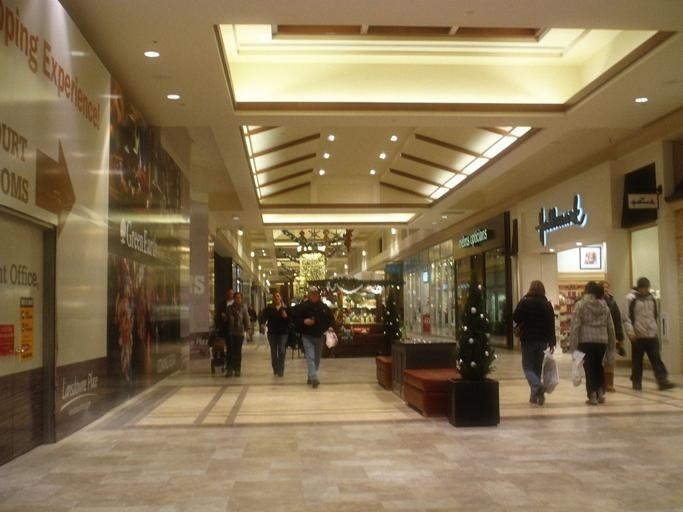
[630,376,642,391]
[585,391,598,405]
[654,373,677,391]
[601,371,616,393]
[597,386,606,403]
[272,363,278,375]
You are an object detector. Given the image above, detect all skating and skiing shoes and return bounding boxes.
[307,380,311,384]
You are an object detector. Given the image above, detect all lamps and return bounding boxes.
[280,230,352,287]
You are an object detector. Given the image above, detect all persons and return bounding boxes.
[512,280,556,406]
[106,255,178,402]
[212,286,335,389]
[568,280,616,407]
[118,103,143,193]
[597,280,625,395]
[623,277,675,391]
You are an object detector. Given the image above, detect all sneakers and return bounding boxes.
[234,370,240,376]
[225,369,232,377]
[528,398,537,404]
[536,386,546,406]
[312,379,320,388]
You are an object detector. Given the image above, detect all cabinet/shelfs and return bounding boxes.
[307,279,407,358]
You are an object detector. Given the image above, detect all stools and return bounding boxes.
[405,368,463,417]
[375,355,394,390]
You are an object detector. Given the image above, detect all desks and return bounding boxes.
[390,339,456,400]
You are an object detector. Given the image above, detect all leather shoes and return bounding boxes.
[277,368,283,377]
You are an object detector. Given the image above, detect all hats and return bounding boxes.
[636,277,650,288]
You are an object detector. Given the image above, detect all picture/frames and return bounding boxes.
[579,246,602,270]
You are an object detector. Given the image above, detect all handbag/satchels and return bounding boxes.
[571,348,586,386]
[322,326,339,350]
[512,322,526,337]
[542,347,559,394]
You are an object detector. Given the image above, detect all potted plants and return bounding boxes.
[447,271,499,427]
[375,288,402,389]
